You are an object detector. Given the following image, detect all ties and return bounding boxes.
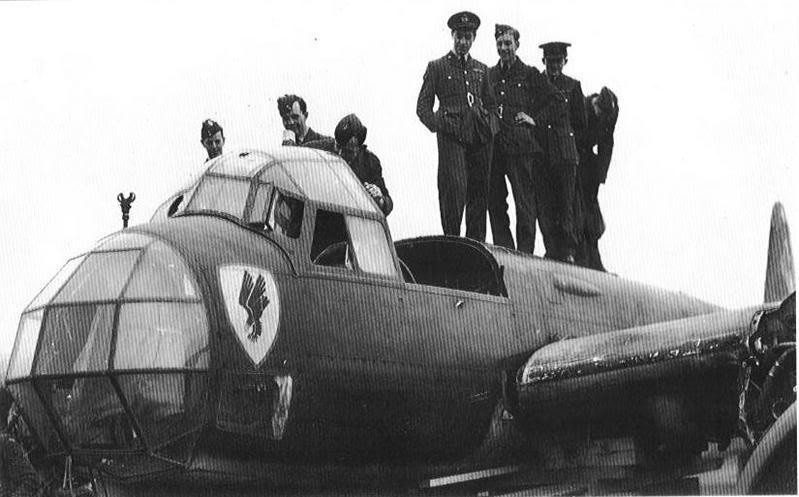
[460,55,466,68]
[502,61,508,73]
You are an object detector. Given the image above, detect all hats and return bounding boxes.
[494,24,512,39]
[598,86,616,112]
[335,113,364,147]
[538,42,571,59]
[201,118,223,140]
[447,12,482,31]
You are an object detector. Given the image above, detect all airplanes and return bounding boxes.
[0,144,798,497]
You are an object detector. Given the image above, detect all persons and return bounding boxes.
[576,88,620,271]
[201,118,226,162]
[276,93,335,147]
[0,385,42,495]
[279,114,397,268]
[417,11,500,241]
[535,40,589,265]
[486,23,568,253]
